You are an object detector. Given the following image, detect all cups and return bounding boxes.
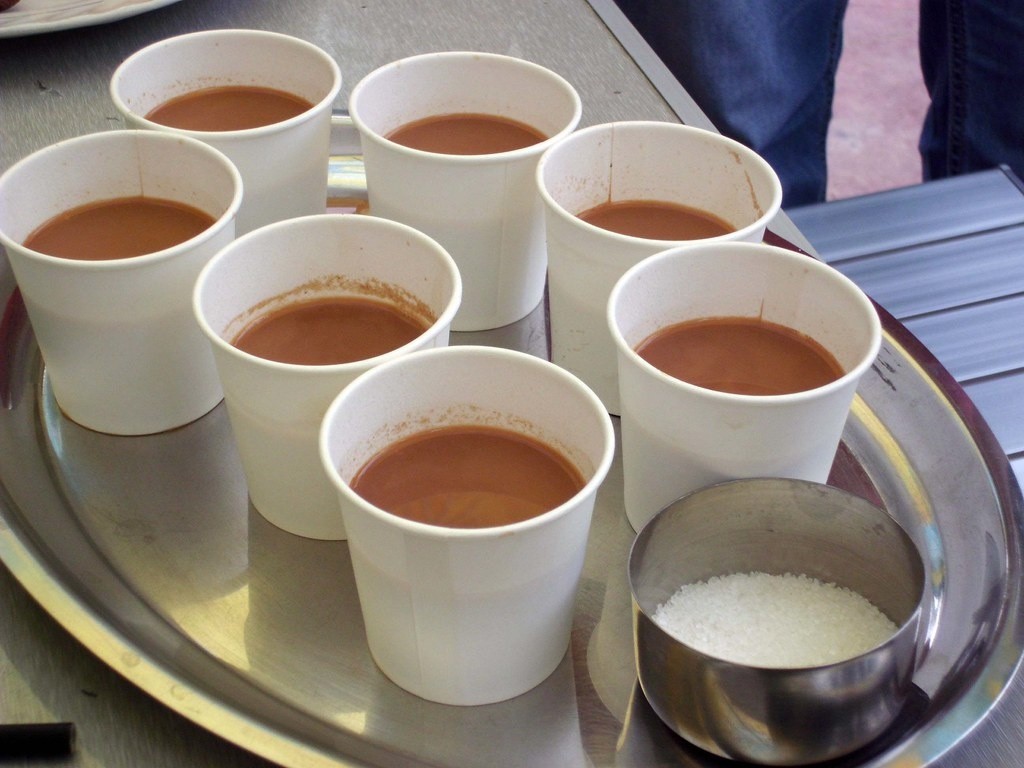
[607,241,882,534]
[348,51,581,330]
[0,129,245,436]
[318,345,616,707]
[109,29,342,239]
[535,121,784,417]
[191,213,463,540]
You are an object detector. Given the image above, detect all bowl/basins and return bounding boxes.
[628,478,926,765]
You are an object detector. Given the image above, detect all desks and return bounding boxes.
[0,1,1024,768]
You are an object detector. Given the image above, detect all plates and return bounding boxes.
[1,0,183,39]
